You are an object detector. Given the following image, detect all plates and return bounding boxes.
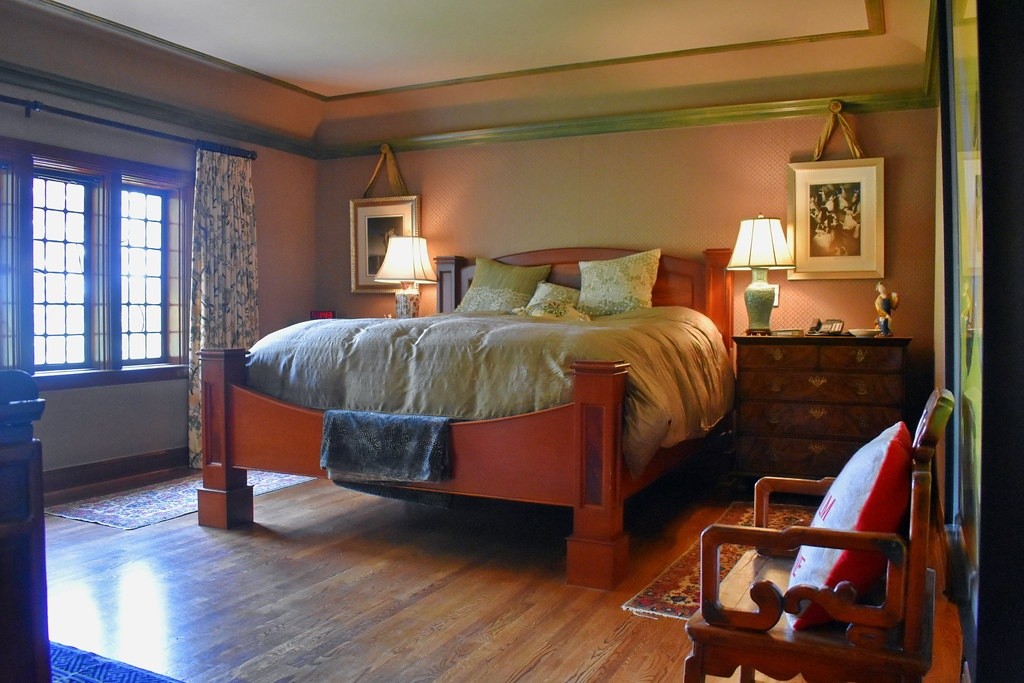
[848,329,882,338]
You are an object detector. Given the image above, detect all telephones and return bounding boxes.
[807,318,845,336]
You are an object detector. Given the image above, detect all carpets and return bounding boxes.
[620,500,818,620]
[50,641,187,683]
[44,470,317,530]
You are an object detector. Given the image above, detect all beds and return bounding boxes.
[194,248,739,588]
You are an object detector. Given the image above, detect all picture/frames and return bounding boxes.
[349,194,421,293]
[786,157,885,281]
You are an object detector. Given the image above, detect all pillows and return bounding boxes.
[453,256,551,315]
[578,248,664,316]
[786,420,912,632]
[511,279,593,322]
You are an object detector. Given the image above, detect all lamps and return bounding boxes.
[725,212,796,337]
[374,235,437,318]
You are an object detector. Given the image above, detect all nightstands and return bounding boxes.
[726,333,913,503]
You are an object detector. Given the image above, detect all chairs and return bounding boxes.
[683,387,955,683]
